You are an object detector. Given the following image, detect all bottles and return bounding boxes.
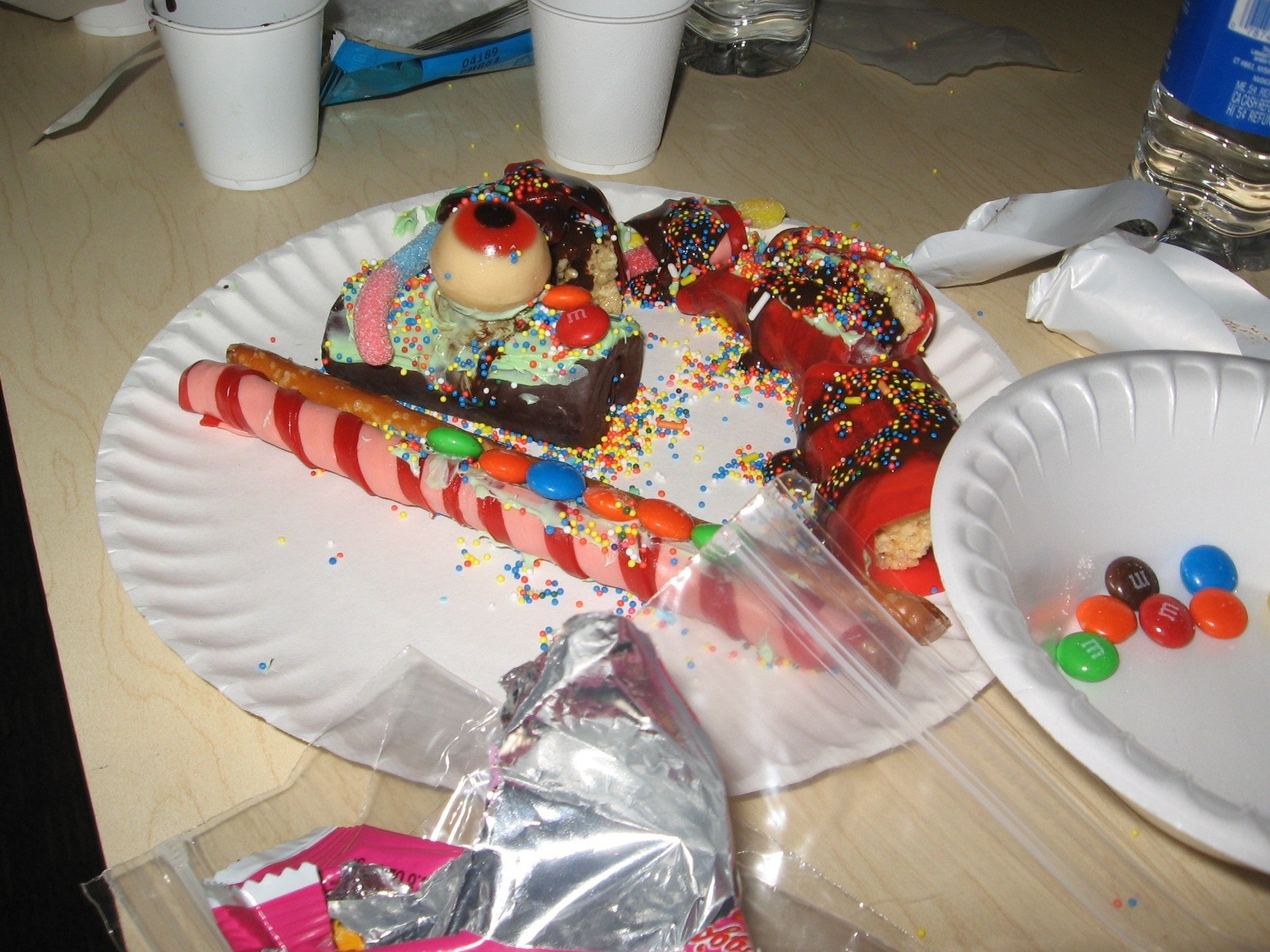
[1127,0,1270,272]
[683,1,815,77]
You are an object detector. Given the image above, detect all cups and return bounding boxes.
[142,0,328,191]
[71,0,151,37]
[529,0,692,175]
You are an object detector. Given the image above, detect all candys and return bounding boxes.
[1052,545,1247,682]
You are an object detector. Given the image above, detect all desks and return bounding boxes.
[0,0,1270,952]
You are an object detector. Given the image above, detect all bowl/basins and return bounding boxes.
[930,349,1270,878]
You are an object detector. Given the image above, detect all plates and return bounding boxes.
[94,180,1023,798]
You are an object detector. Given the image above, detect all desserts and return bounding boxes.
[180,160,969,686]
